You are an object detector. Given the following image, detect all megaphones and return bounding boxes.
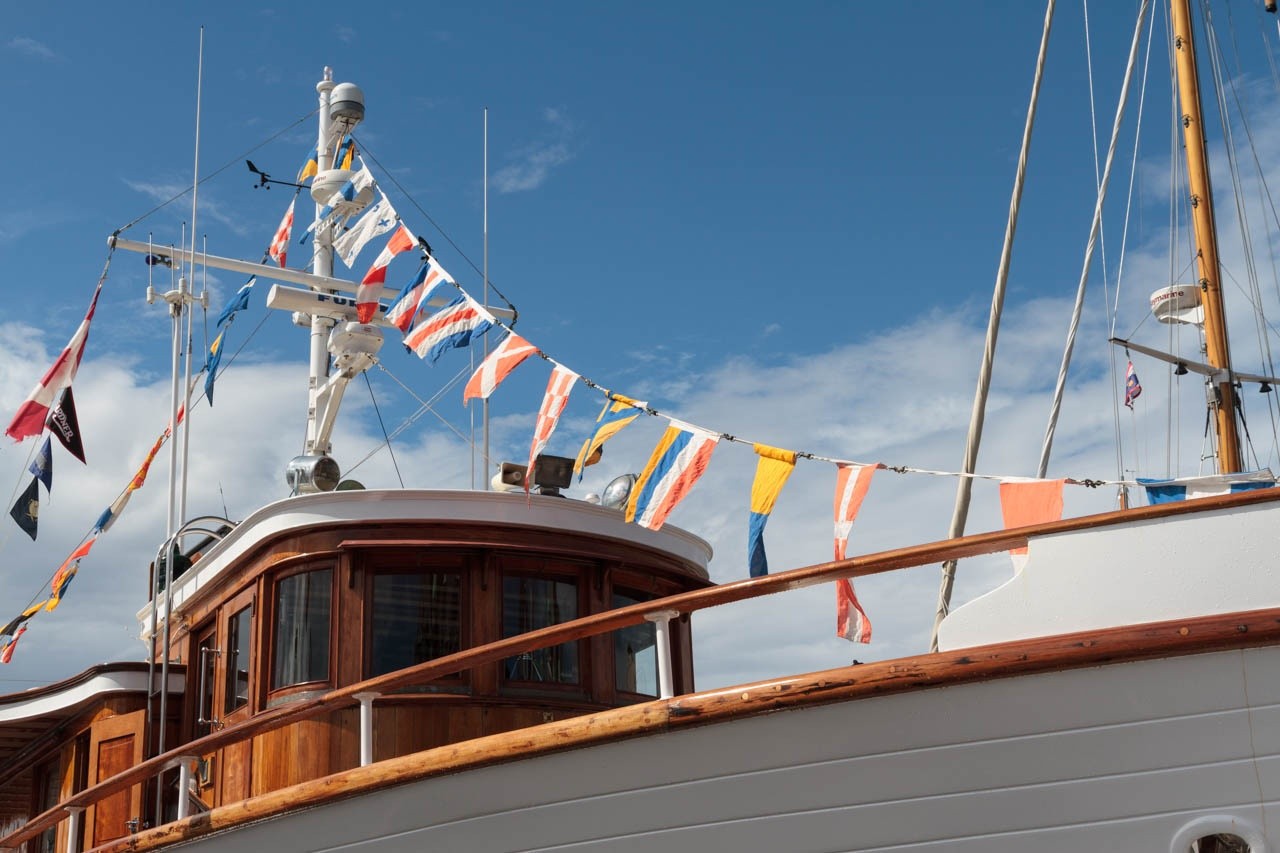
[492,462,527,492]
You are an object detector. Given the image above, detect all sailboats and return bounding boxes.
[0,1,1279,853]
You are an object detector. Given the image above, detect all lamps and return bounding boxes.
[601,473,638,510]
[534,452,576,499]
[286,455,340,497]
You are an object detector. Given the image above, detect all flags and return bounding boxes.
[298,157,1277,646]
[0,132,359,674]
